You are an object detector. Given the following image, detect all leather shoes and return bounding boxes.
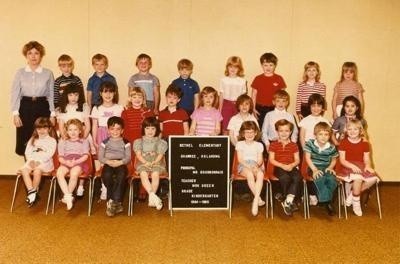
[328,205,335,215]
[60,188,85,209]
[25,190,40,208]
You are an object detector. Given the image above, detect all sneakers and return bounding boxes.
[147,196,163,210]
[252,197,265,216]
[101,190,123,217]
[346,192,363,216]
[274,193,318,216]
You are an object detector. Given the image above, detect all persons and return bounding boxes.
[10,40,380,219]
